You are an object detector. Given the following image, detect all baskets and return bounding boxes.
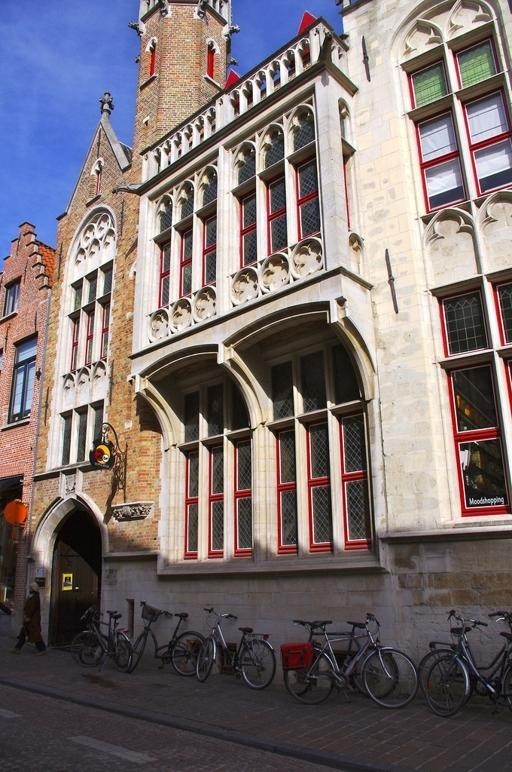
[142,605,160,622]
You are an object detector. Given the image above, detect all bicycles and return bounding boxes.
[195,605,277,690]
[129,597,209,678]
[280,608,512,720]
[71,604,134,677]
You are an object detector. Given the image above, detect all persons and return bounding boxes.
[9,582,47,657]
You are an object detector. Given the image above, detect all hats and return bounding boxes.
[30,582,40,592]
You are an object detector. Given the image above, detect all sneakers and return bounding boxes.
[8,640,47,658]
